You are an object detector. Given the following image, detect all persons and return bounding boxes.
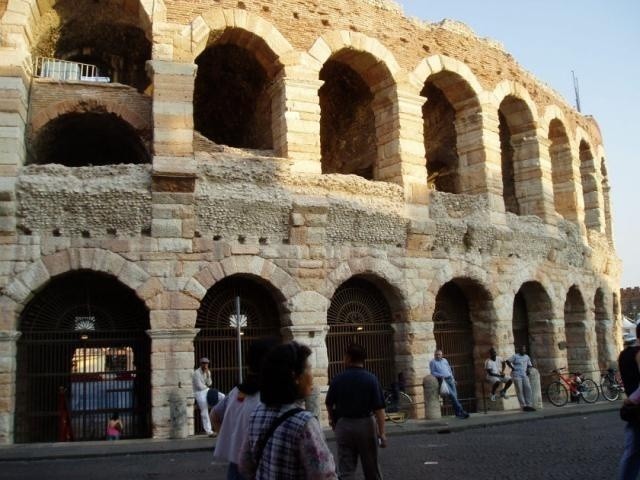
[325,343,389,480]
[236,340,340,480]
[484,347,512,402]
[209,338,284,479]
[622,350,640,426]
[429,348,470,420]
[390,371,407,405]
[568,369,585,404]
[505,343,537,412]
[192,355,226,438]
[106,409,124,441]
[614,320,640,480]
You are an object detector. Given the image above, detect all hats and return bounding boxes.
[199,357,210,364]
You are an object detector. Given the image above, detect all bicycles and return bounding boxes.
[546,367,625,407]
[383,391,413,423]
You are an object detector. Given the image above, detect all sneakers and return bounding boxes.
[488,390,507,402]
[207,433,217,438]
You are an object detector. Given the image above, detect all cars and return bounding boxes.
[622,317,637,346]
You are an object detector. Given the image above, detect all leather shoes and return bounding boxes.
[523,406,536,412]
